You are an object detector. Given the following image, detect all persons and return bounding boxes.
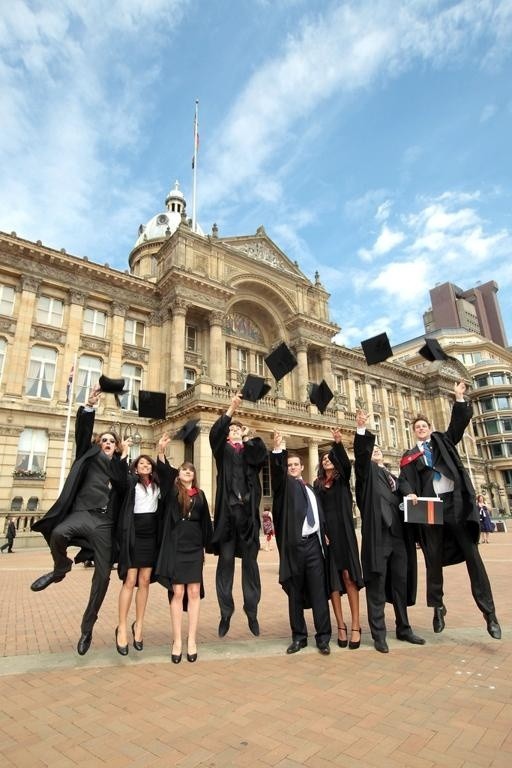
[111,433,168,656]
[261,504,274,552]
[208,387,268,638]
[0,515,16,553]
[271,425,332,655]
[30,385,133,657]
[157,431,214,664]
[313,423,367,650]
[398,377,502,640]
[477,495,495,543]
[353,406,427,654]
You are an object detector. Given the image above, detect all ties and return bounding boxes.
[302,484,315,528]
[421,441,442,481]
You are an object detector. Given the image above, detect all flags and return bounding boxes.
[66,353,77,403]
[191,109,200,169]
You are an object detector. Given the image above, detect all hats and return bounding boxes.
[362,333,393,366]
[138,390,167,419]
[309,380,333,414]
[419,338,448,362]
[95,375,128,407]
[265,343,297,382]
[174,419,201,444]
[239,374,270,403]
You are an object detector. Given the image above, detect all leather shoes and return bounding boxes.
[287,623,390,654]
[484,611,501,640]
[432,605,447,633]
[171,639,183,664]
[186,638,199,663]
[397,629,426,644]
[115,626,130,655]
[250,616,260,636]
[132,622,144,650]
[219,614,230,638]
[30,571,66,592]
[77,631,90,655]
